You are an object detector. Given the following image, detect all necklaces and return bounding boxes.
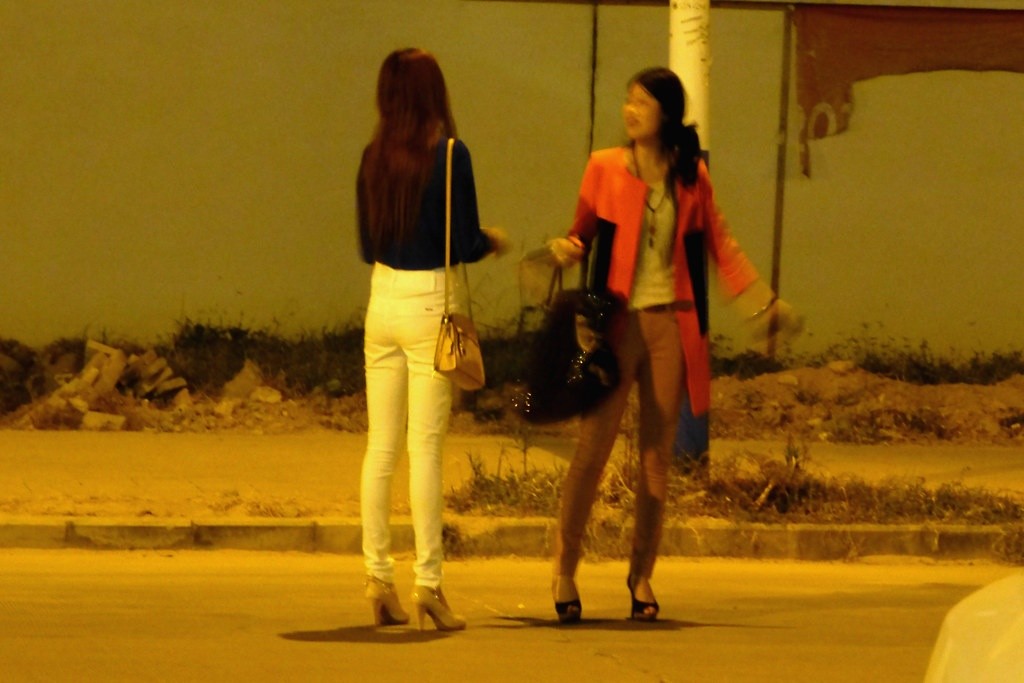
[630,144,672,248]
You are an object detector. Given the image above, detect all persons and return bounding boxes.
[543,65,802,625]
[354,44,513,632]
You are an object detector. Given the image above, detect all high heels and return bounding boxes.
[412,585,467,632]
[366,577,409,627]
[551,578,582,624]
[627,574,660,621]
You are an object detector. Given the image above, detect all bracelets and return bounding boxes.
[740,293,779,324]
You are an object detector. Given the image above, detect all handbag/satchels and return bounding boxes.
[434,311,483,390]
[507,232,623,424]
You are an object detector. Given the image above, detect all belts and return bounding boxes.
[644,301,692,314]
